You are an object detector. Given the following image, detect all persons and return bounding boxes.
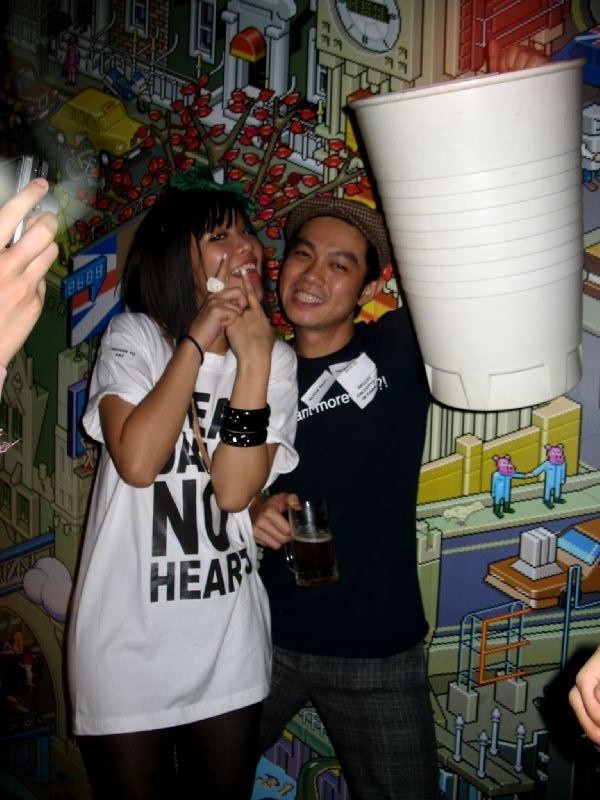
[569,646,600,744]
[0,179,60,400]
[256,196,448,800]
[67,187,301,800]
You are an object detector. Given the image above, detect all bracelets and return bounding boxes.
[217,401,272,447]
[187,334,204,367]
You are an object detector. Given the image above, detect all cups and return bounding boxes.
[348,58,589,414]
[281,497,343,587]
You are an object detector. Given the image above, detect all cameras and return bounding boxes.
[6,156,59,251]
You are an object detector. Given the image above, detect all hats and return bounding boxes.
[282,196,390,274]
